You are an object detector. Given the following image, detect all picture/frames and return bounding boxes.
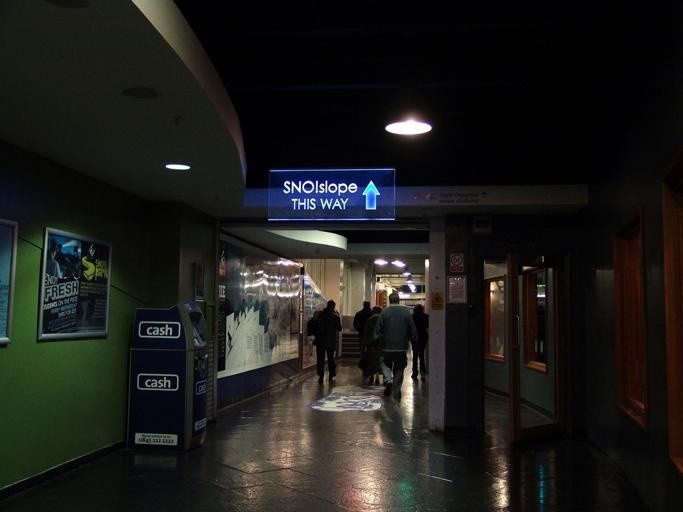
[0,219,113,346]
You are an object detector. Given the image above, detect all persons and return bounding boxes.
[48,239,63,279]
[308,300,344,386]
[374,293,421,399]
[358,306,384,389]
[410,303,428,382]
[354,300,374,352]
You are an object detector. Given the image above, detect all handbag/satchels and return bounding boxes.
[306,311,320,336]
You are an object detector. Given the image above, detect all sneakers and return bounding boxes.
[384,380,392,396]
